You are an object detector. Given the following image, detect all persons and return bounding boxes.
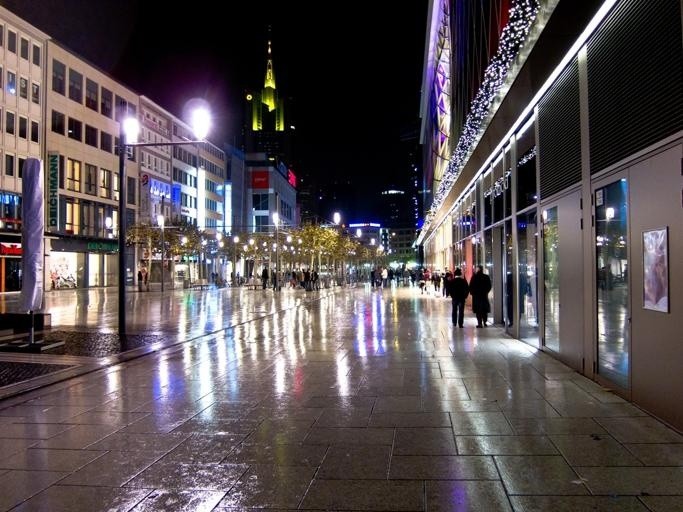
[237,271,240,282]
[469,265,494,328]
[208,272,218,283]
[138,271,150,291]
[261,267,320,291]
[449,268,470,328]
[506,266,527,327]
[367,264,452,297]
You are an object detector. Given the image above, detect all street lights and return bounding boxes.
[117,108,211,351]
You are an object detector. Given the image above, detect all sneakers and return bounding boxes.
[453,322,487,328]
